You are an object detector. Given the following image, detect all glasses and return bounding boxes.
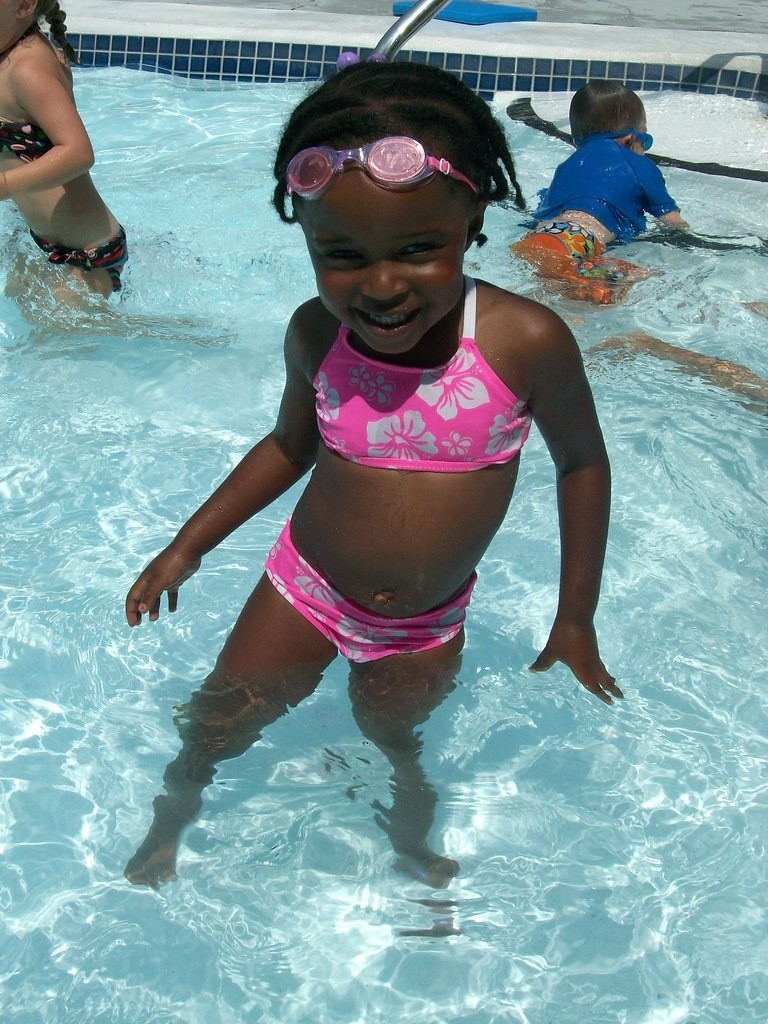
[285,135,437,200]
[633,130,653,151]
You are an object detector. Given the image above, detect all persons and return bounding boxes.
[125,61,627,708]
[544,78,680,233]
[0,0,129,301]
